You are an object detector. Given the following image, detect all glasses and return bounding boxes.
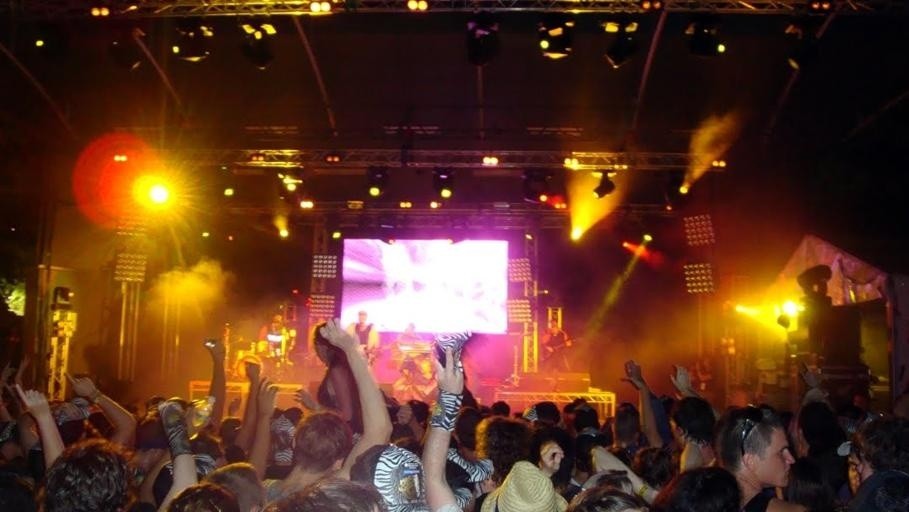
[738,403,775,456]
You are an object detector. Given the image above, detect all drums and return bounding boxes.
[237,355,263,381]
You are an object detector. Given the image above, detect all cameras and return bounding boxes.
[203,336,216,350]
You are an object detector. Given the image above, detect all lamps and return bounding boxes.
[1,0,835,246]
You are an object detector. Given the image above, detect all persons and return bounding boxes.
[4,309,909,511]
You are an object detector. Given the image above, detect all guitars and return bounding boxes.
[364,344,393,366]
[541,341,576,362]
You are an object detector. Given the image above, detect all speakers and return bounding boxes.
[555,370,592,390]
[518,370,554,390]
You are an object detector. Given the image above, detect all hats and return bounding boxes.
[478,458,572,512]
